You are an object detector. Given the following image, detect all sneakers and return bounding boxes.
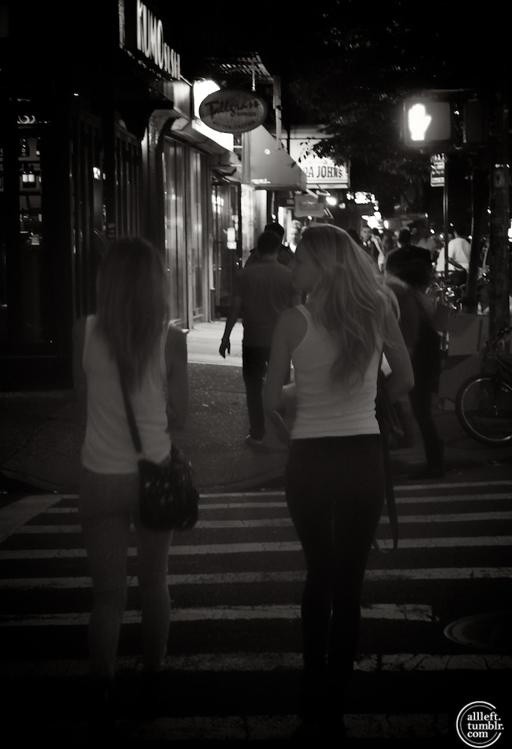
[246,433,266,447]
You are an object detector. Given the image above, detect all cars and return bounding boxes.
[478,232,512,317]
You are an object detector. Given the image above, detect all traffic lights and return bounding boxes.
[399,88,456,148]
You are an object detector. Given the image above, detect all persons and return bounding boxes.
[218,219,473,709]
[67,235,192,704]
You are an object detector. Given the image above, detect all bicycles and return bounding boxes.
[454,325,512,448]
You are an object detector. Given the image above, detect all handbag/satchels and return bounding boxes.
[134,445,198,532]
